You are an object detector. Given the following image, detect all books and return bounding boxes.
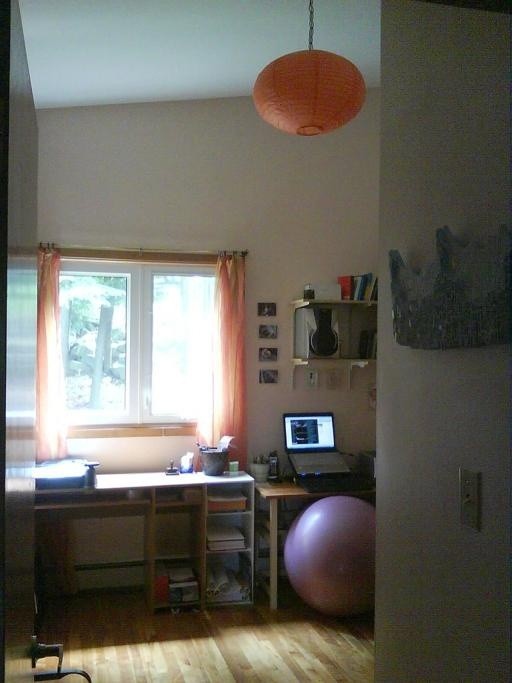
[359,329,378,360]
[165,565,200,603]
[336,273,378,301]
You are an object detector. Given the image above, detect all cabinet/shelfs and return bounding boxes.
[286,298,377,391]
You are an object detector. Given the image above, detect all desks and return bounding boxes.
[254,480,376,611]
[33,468,255,617]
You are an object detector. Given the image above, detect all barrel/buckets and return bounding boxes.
[199,446,230,477]
[250,463,270,483]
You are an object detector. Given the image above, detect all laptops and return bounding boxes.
[283,412,351,476]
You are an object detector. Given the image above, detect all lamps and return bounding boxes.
[251,0,365,135]
